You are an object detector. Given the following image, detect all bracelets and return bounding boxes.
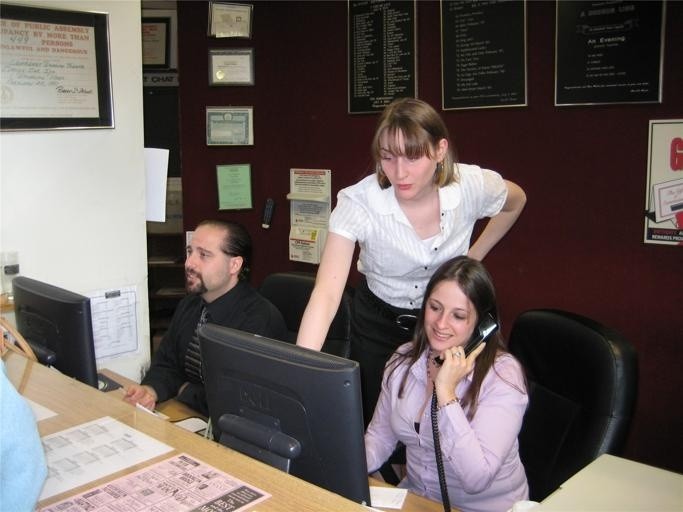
[437,396,459,408]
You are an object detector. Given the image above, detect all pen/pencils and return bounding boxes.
[119,387,156,414]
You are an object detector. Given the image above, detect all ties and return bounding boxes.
[184,306,208,385]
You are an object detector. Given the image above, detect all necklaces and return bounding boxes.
[426,348,432,381]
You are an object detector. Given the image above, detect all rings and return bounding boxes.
[452,351,461,359]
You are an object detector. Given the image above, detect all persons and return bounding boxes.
[292,97,529,459]
[115,218,283,416]
[359,254,530,512]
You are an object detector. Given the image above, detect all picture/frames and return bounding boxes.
[1,1,115,133]
[205,1,255,146]
[642,119,683,249]
[141,17,170,68]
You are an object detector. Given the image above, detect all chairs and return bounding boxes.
[257,272,357,360]
[506,309,641,502]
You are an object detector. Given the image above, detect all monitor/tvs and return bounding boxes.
[11,277,99,390]
[198,323,371,507]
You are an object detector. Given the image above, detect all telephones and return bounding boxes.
[432,312,499,367]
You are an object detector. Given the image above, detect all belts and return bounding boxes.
[395,314,418,332]
